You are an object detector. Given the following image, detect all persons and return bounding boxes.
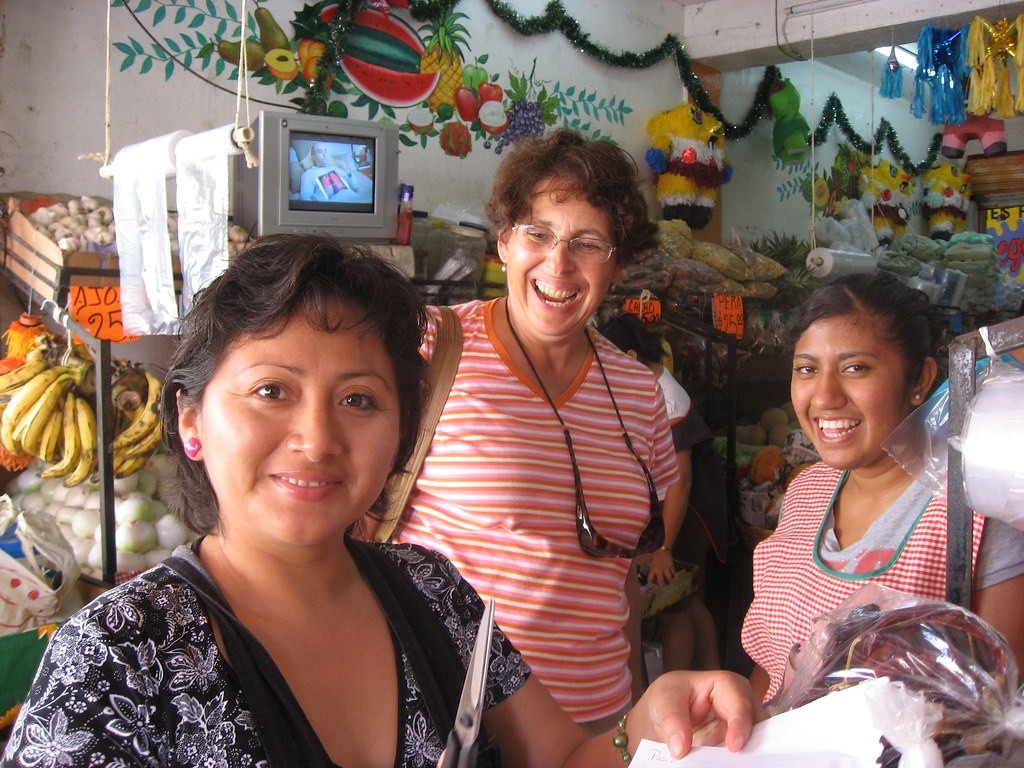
[350,126,681,735]
[299,143,363,202]
[0,235,769,768]
[596,311,736,702]
[740,273,1024,705]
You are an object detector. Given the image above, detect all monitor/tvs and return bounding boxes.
[230,110,399,243]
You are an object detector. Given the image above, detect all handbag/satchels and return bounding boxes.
[0,493,84,637]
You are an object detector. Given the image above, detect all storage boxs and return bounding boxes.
[0,191,185,307]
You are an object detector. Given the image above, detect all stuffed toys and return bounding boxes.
[769,78,811,165]
[941,76,1007,158]
[923,161,974,241]
[646,103,733,230]
[858,157,914,241]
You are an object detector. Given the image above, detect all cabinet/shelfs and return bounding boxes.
[0,266,219,605]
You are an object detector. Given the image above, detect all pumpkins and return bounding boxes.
[749,443,815,531]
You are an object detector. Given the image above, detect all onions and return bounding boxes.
[5,450,197,579]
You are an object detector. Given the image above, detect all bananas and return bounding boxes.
[0,334,170,487]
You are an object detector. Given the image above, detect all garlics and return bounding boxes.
[26,195,248,256]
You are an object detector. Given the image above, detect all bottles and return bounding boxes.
[391,184,509,307]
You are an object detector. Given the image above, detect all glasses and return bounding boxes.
[510,224,617,264]
[564,429,665,558]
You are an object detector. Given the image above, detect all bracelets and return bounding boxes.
[613,713,632,765]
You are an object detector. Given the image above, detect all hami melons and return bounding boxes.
[734,400,803,448]
